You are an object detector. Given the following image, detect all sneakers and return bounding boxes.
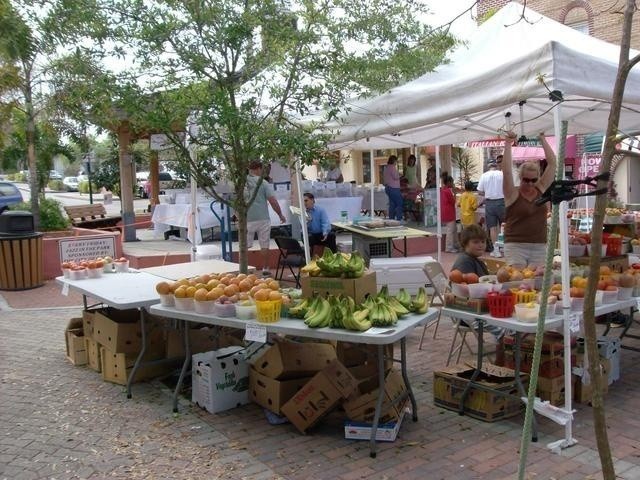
[401,219,409,225]
[262,272,273,278]
[489,251,502,258]
[445,248,458,253]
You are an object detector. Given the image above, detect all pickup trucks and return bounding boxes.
[158,171,187,189]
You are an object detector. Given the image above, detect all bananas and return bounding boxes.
[301,247,365,278]
[473,390,487,410]
[435,381,448,397]
[289,284,429,332]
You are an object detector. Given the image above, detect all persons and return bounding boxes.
[455,181,478,231]
[383,155,409,225]
[502,129,557,267]
[440,172,464,253]
[496,155,520,187]
[326,161,343,183]
[403,155,421,190]
[424,156,436,188]
[239,161,286,279]
[301,192,337,265]
[476,162,506,258]
[264,164,273,184]
[449,223,516,345]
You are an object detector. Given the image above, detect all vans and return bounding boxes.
[0,182,24,214]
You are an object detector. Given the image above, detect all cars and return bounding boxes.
[63,176,82,192]
[48,169,63,181]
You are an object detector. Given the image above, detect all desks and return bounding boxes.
[330,217,436,258]
[138,257,256,284]
[353,190,389,218]
[156,195,363,246]
[55,262,180,400]
[441,295,640,450]
[150,307,440,458]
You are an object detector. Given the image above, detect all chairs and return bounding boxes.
[418,262,485,355]
[275,235,306,289]
[280,247,305,288]
[445,286,499,367]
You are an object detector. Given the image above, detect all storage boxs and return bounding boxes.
[83,306,121,338]
[192,346,254,415]
[575,254,629,274]
[188,323,233,354]
[575,335,621,386]
[495,330,577,378]
[574,353,610,404]
[433,358,532,423]
[94,307,164,353]
[64,317,86,365]
[100,346,166,385]
[219,328,246,349]
[343,367,411,424]
[444,292,489,315]
[249,366,314,417]
[280,357,360,432]
[345,394,410,442]
[164,325,187,358]
[248,341,337,379]
[528,373,575,407]
[302,268,377,307]
[84,336,105,372]
[368,256,438,296]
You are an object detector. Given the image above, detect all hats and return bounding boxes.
[486,158,496,163]
[428,156,435,161]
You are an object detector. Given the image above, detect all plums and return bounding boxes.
[239,292,248,300]
[73,268,77,270]
[224,301,232,304]
[77,267,81,270]
[228,297,233,301]
[232,294,238,302]
[80,266,85,269]
[219,295,227,304]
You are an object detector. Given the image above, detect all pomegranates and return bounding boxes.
[600,274,609,280]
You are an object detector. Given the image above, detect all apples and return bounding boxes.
[459,282,469,297]
[632,263,640,270]
[519,289,529,302]
[466,273,478,284]
[115,259,119,262]
[547,295,557,304]
[598,277,606,290]
[450,269,462,283]
[601,266,610,274]
[97,258,102,261]
[618,271,635,287]
[101,259,108,263]
[488,288,499,303]
[527,303,535,309]
[120,257,126,262]
[552,290,560,296]
[497,269,511,282]
[105,256,112,262]
[633,272,640,284]
[498,288,512,304]
[249,299,255,306]
[623,266,633,275]
[552,283,562,291]
[519,283,531,291]
[609,272,619,285]
[240,299,249,306]
[631,239,638,245]
[510,269,523,280]
[606,285,617,290]
[505,264,516,273]
[559,290,564,299]
[462,273,467,281]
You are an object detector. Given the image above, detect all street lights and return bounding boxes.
[81,150,97,204]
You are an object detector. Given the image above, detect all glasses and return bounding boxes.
[521,176,539,183]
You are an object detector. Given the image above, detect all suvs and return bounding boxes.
[134,175,149,198]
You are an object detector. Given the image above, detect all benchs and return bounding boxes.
[63,203,122,233]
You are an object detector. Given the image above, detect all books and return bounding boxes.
[290,205,309,218]
